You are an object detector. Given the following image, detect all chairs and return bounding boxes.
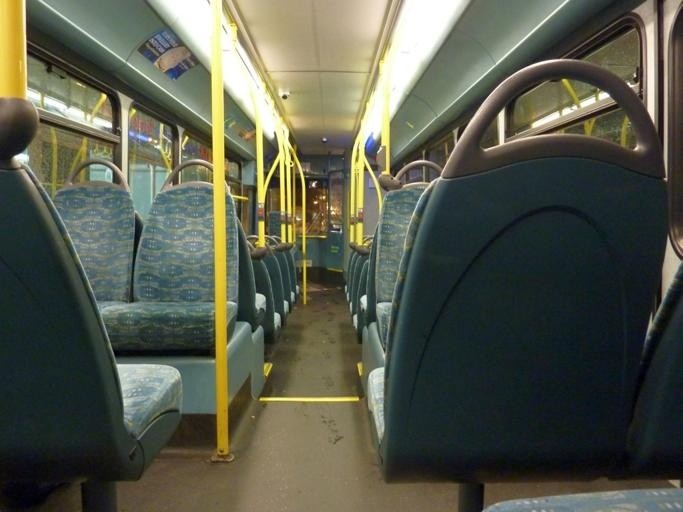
[0,95,305,509]
[343,57,682,512]
